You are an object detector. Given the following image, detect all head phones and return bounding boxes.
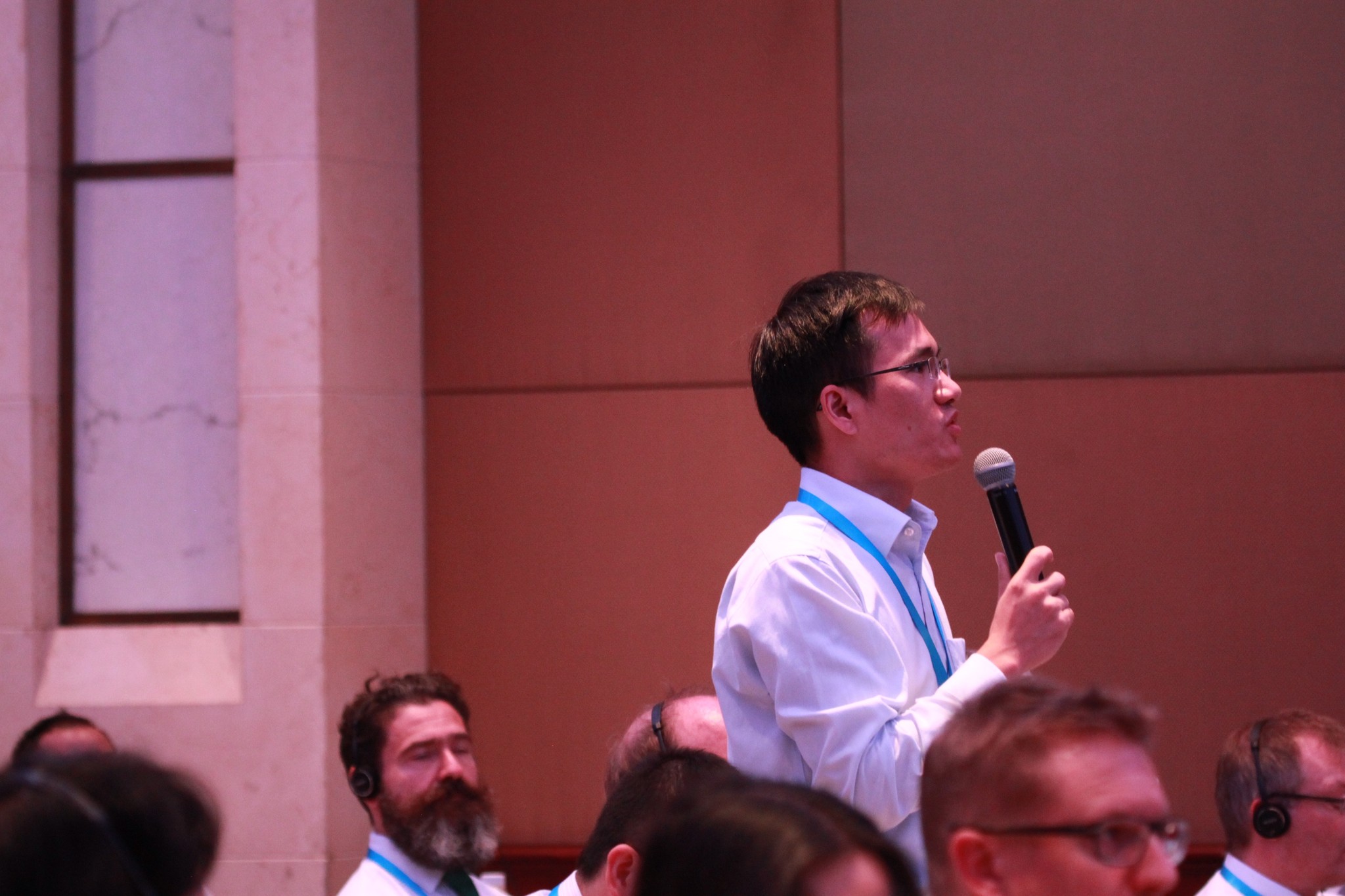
[344,710,378,801]
[1248,719,1290,840]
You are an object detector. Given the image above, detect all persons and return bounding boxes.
[604,694,728,797]
[15,712,116,752]
[1196,712,1345,896]
[336,667,511,896]
[712,269,1073,888]
[0,747,221,896]
[634,779,923,896]
[917,676,1189,896]
[525,748,746,896]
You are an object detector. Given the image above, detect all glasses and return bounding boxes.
[816,357,951,412]
[961,817,1190,868]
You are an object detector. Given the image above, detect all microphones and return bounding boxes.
[973,446,1044,582]
